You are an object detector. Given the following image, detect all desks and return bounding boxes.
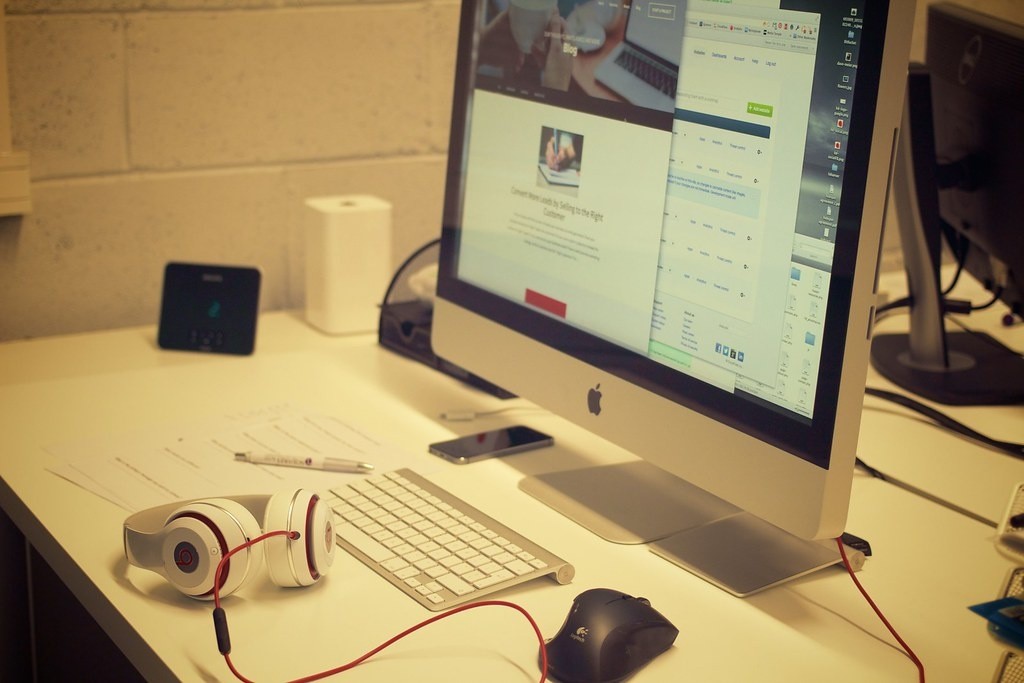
[1,264,1024,681]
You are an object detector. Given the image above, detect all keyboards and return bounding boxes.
[318,467,575,613]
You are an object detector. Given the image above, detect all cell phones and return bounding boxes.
[429,426,553,465]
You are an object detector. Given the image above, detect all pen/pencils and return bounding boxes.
[230,449,375,477]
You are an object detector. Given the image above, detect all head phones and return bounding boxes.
[124,489,338,601]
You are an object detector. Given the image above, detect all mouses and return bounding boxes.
[539,589,679,683]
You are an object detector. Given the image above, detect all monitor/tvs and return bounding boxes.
[870,1,1023,405]
[431,0,916,597]
